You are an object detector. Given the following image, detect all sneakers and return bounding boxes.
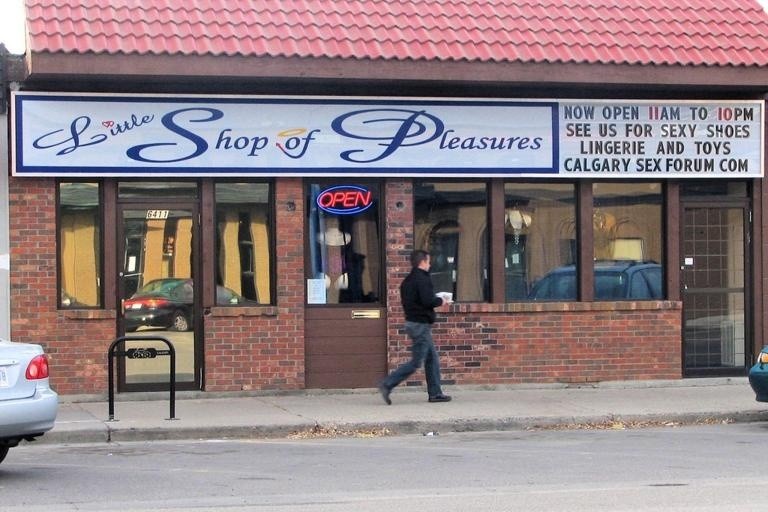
[430,394,452,402]
[377,382,393,404]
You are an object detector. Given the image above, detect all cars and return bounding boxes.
[684,309,749,368]
[749,345,768,403]
[525,258,664,301]
[122,278,259,332]
[60,290,87,307]
[0,338,60,465]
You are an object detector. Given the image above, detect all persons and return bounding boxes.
[379,250,452,402]
